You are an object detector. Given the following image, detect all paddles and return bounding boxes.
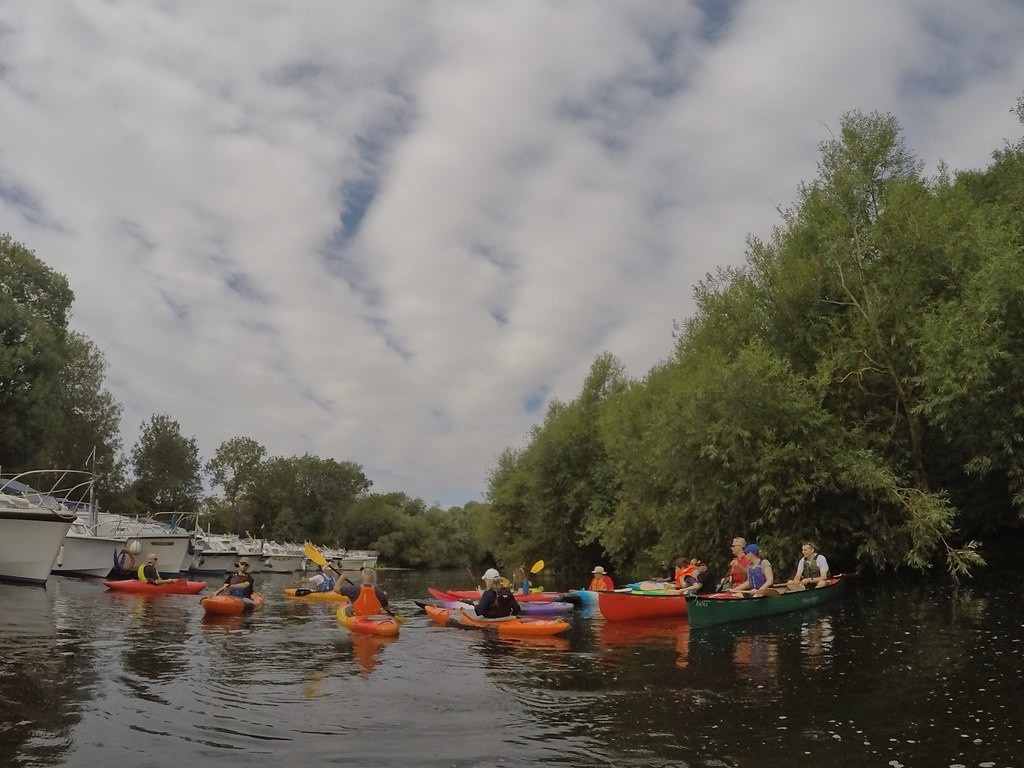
[199,574,241,603]
[717,565,731,593]
[426,586,549,621]
[722,588,780,597]
[466,565,478,589]
[774,576,822,587]
[640,581,667,591]
[304,541,406,623]
[527,560,545,574]
[593,587,632,593]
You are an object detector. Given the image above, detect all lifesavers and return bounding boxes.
[120,549,135,571]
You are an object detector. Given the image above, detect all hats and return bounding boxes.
[238,557,251,565]
[482,568,500,580]
[322,562,333,572]
[591,566,607,574]
[742,544,760,554]
[694,559,708,566]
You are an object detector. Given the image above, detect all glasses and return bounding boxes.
[240,563,248,566]
[731,544,741,548]
[154,558,157,561]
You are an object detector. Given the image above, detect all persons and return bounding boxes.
[334,568,389,616]
[721,537,750,588]
[474,568,520,619]
[223,557,255,596]
[514,565,525,590]
[589,566,614,591]
[736,544,773,598]
[292,565,335,592]
[787,542,829,590]
[659,558,716,594]
[138,553,161,583]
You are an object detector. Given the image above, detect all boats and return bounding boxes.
[337,603,400,636]
[565,587,634,605]
[596,590,725,622]
[48,493,379,580]
[427,587,577,613]
[285,587,351,605]
[684,573,849,628]
[0,468,100,585]
[200,589,265,613]
[411,600,570,636]
[100,576,209,594]
